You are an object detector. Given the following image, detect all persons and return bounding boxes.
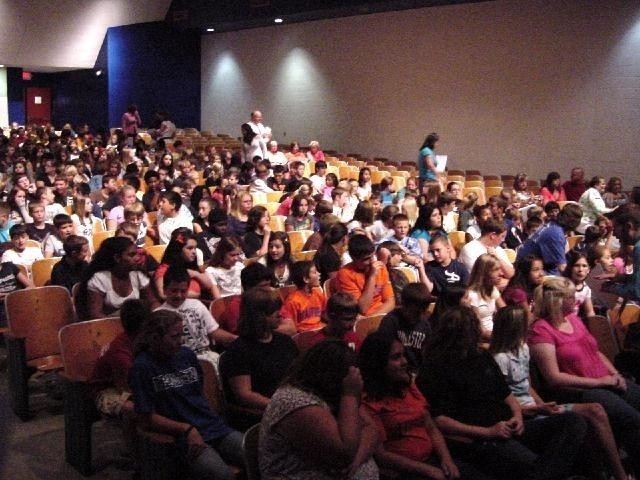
[1,120,639,480]
[120,104,142,134]
[241,111,272,162]
